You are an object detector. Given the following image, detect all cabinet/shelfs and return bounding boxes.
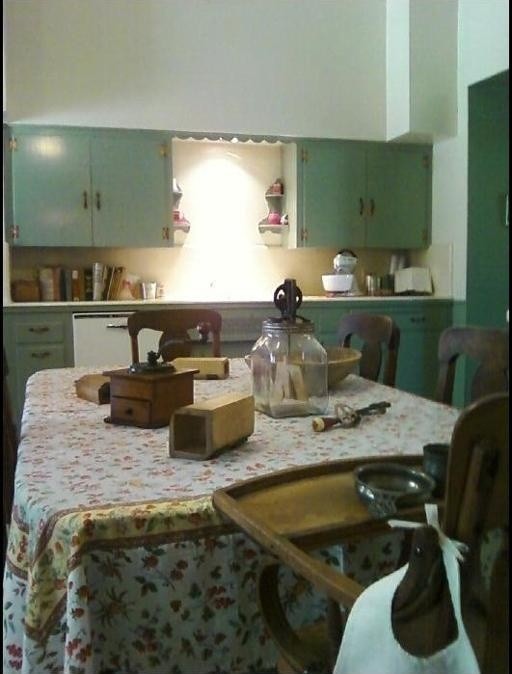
[260,195,285,229]
[301,305,455,406]
[4,125,171,249]
[3,308,73,427]
[285,136,433,251]
[171,191,189,230]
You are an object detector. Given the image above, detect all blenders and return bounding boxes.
[322,248,365,298]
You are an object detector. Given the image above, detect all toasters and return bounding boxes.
[392,265,434,296]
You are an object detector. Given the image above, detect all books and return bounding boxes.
[38,262,125,301]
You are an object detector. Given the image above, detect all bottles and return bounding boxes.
[248,320,330,417]
[268,211,280,225]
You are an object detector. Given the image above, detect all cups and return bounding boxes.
[141,281,157,301]
[421,443,450,499]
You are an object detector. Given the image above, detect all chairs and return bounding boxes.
[127,307,224,361]
[435,325,510,407]
[334,313,401,386]
[208,393,509,672]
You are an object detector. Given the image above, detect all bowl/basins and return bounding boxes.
[244,345,362,404]
[352,462,436,519]
